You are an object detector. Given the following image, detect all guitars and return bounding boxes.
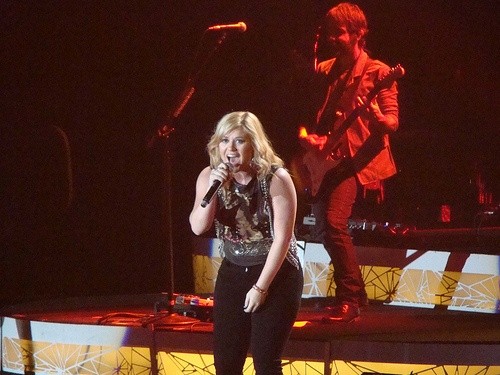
[285,64,406,205]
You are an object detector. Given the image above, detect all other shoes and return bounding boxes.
[321,302,361,326]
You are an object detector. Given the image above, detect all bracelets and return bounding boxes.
[253,284,268,295]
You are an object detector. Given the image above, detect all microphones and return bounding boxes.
[208,22,246,32]
[201,162,233,208]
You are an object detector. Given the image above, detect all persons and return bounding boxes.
[289,3,400,324]
[190,111,304,375]
